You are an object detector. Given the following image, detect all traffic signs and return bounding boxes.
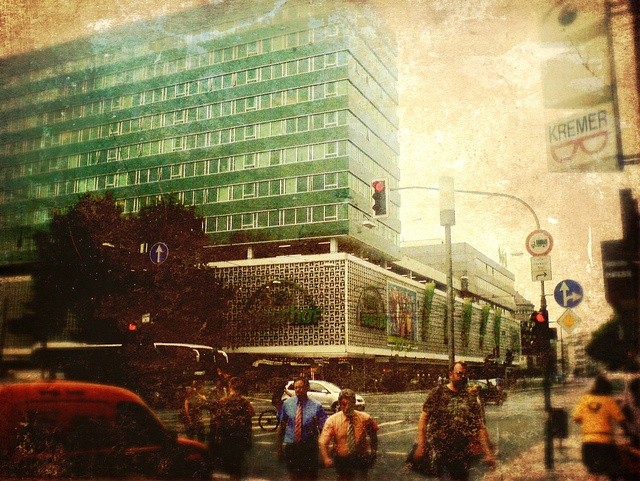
[530,255,552,282]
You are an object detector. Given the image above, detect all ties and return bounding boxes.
[347,417,355,454]
[295,401,302,443]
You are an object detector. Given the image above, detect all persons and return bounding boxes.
[620,375,640,481]
[275,376,327,480]
[212,376,256,481]
[318,388,380,481]
[571,373,630,481]
[406,360,497,481]
[180,378,214,442]
[272,376,293,419]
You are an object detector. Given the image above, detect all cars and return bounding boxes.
[0,377,217,480]
[470,379,512,410]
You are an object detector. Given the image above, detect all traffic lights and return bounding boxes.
[530,309,550,353]
[371,176,389,219]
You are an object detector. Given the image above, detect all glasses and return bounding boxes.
[452,371,471,377]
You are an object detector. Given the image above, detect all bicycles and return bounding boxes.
[258,401,286,434]
